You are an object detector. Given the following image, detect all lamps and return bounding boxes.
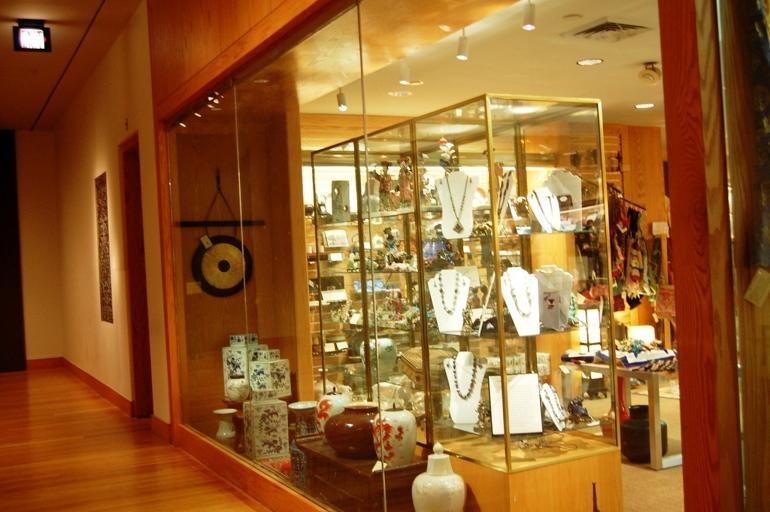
[334,88,352,113]
[522,0,537,32]
[457,27,470,60]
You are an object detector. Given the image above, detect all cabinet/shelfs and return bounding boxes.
[310,92,622,512]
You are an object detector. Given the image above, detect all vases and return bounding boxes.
[311,384,417,471]
[290,398,320,489]
[213,335,292,477]
[621,404,668,464]
[412,440,466,510]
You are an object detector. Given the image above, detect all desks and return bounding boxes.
[560,358,682,470]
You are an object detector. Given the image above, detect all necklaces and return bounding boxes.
[445,175,468,234]
[440,272,459,315]
[545,386,566,422]
[506,272,532,318]
[453,355,477,400]
[533,190,553,224]
